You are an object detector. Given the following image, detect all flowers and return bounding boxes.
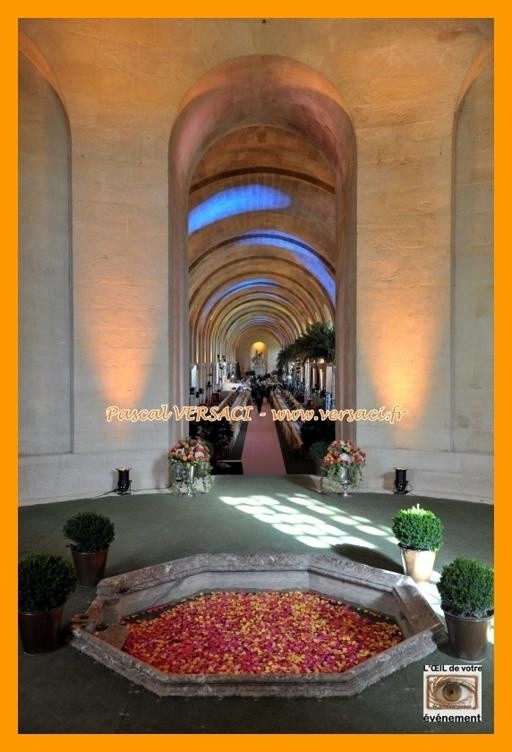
[171,434,215,497]
[320,440,366,497]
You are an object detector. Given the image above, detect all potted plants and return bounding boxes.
[393,504,494,661]
[18,512,115,655]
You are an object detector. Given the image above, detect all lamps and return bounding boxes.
[115,466,133,494]
[392,467,410,495]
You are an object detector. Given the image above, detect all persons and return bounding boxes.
[242,368,332,422]
